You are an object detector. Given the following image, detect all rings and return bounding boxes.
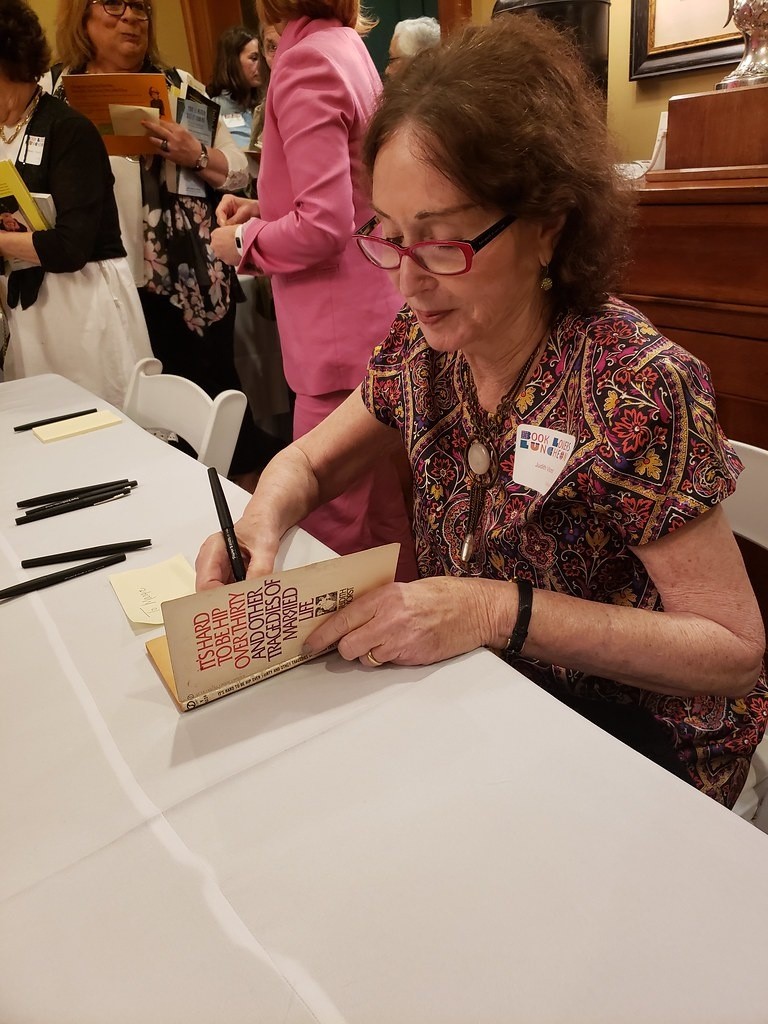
[160,140,167,152]
[367,651,383,666]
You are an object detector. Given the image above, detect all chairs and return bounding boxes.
[124,358,248,479]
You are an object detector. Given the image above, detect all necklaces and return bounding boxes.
[1,86,43,144]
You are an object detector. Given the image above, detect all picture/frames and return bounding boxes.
[628,0,745,81]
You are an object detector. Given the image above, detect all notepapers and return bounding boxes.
[30,410,125,443]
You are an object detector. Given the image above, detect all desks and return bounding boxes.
[0,369,768,1024]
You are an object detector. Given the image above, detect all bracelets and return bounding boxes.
[234,225,244,256]
[504,577,533,655]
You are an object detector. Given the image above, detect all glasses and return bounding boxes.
[353,213,519,275]
[260,43,278,57]
[93,0,153,21]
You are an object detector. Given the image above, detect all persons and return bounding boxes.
[35,0,287,468]
[195,44,768,811]
[209,0,420,583]
[0,0,178,444]
[384,16,441,83]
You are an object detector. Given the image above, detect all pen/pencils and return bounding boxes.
[207,467,246,582]
[14,408,97,431]
[17,479,128,508]
[15,486,131,525]
[21,539,152,569]
[0,552,127,599]
[25,480,138,515]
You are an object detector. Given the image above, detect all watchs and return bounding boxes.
[191,143,208,172]
